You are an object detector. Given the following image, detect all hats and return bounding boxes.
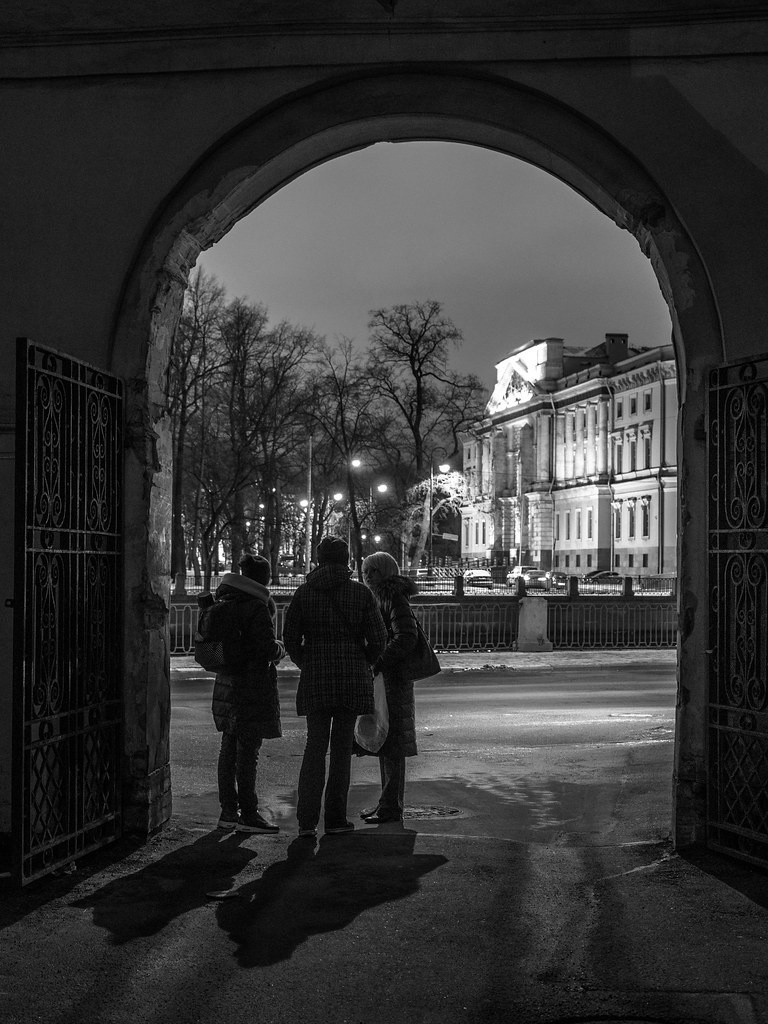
[237,553,270,586]
[316,535,349,566]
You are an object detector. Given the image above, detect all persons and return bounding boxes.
[352,552,416,823]
[212,554,285,832]
[284,537,388,835]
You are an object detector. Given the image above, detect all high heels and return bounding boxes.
[361,803,402,824]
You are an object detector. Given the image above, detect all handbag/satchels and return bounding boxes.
[408,624,442,681]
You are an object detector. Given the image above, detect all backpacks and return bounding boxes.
[194,594,244,673]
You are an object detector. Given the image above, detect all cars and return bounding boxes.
[589,571,623,585]
[462,570,494,590]
[409,568,436,587]
[546,571,569,589]
[580,570,604,584]
[505,566,538,587]
[523,570,547,591]
[487,566,504,583]
[278,555,306,568]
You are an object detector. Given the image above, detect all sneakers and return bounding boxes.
[324,821,355,833]
[298,823,318,836]
[216,809,279,834]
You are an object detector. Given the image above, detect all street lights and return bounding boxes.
[369,478,387,557]
[426,446,451,586]
[347,457,360,571]
